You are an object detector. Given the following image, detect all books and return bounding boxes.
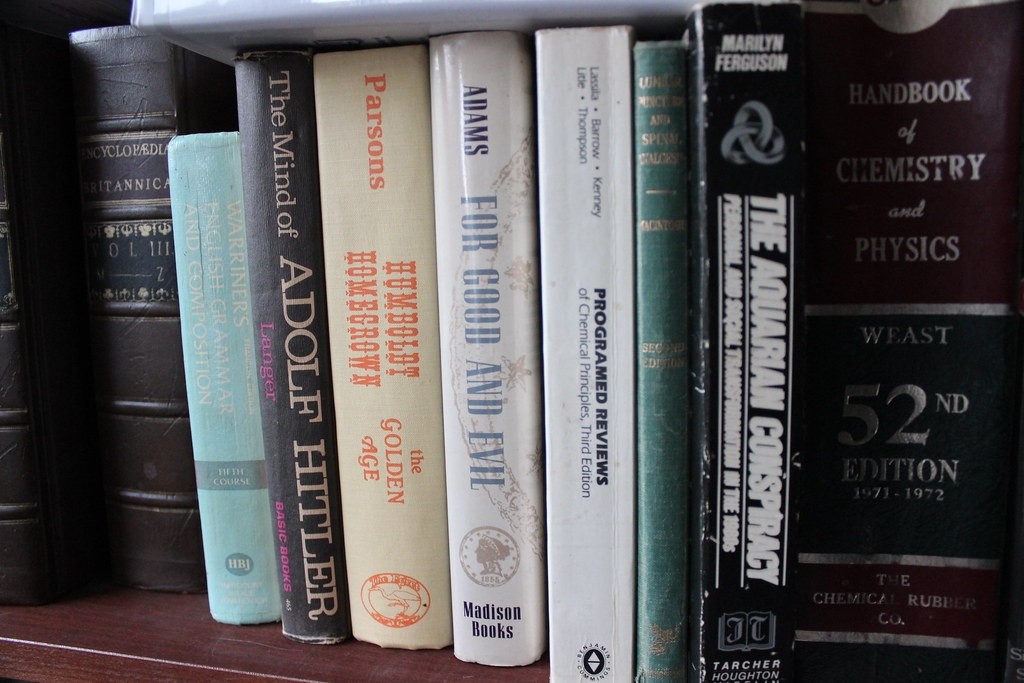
[0,0,1024,683]
